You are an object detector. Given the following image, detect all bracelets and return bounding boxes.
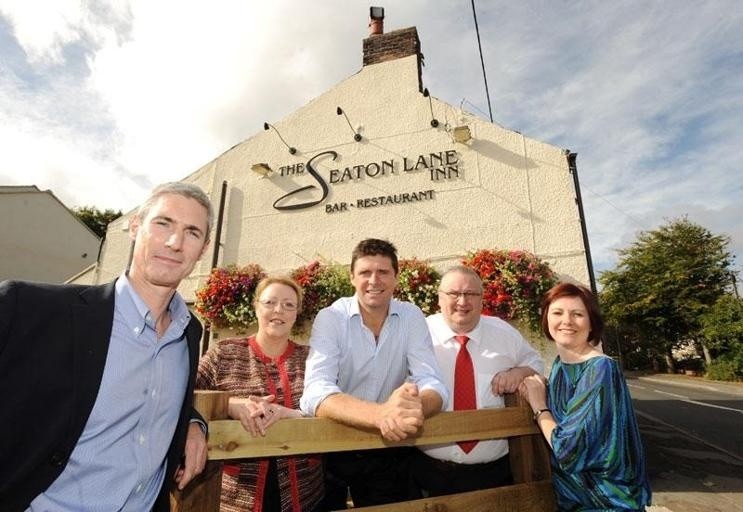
[532,408,550,423]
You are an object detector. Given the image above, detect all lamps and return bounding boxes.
[263,121,297,157]
[421,88,440,128]
[334,105,362,145]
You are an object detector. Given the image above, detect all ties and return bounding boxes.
[453,335,479,453]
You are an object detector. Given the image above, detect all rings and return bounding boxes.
[269,410,273,414]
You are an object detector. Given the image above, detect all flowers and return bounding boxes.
[392,257,444,320]
[193,262,270,335]
[288,259,357,342]
[455,245,561,353]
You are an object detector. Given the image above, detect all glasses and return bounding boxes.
[439,289,482,300]
[257,299,301,311]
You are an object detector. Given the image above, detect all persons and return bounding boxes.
[407,264,547,499]
[300,237,452,512]
[0,181,210,511]
[194,275,328,511]
[516,281,653,512]
